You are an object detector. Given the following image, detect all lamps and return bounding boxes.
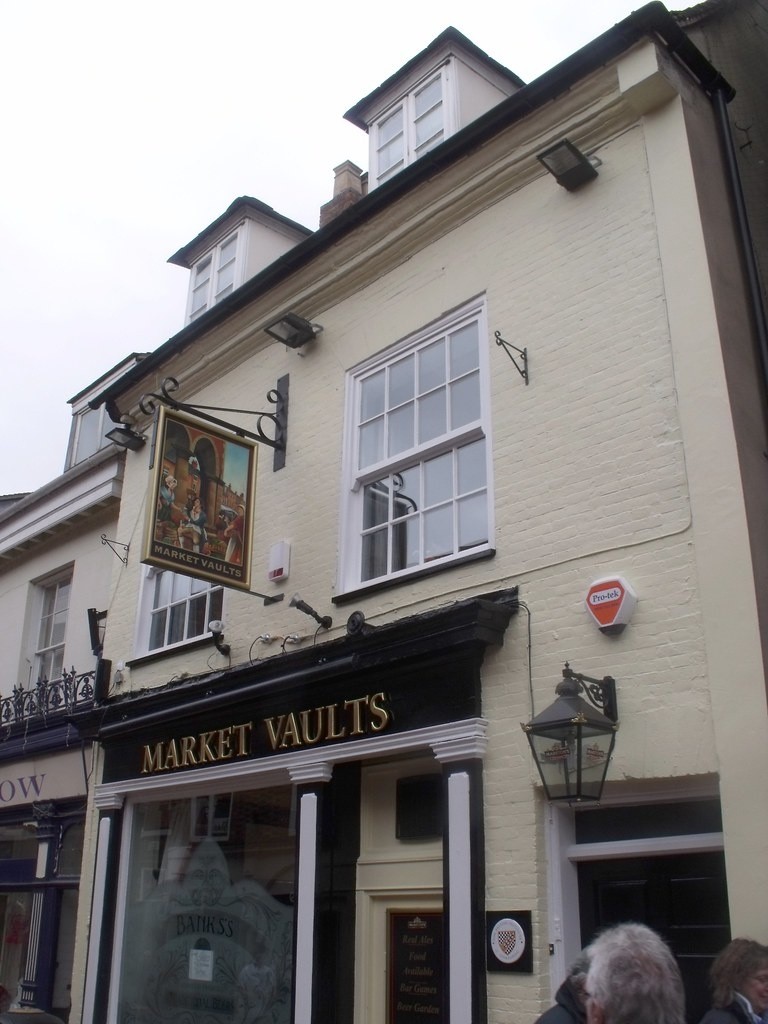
[535,137,601,191]
[264,312,323,350]
[519,661,618,804]
[209,620,231,656]
[105,428,148,451]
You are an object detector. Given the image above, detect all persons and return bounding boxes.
[700,938,768,1024]
[235,949,276,1024]
[534,945,596,1024]
[585,924,687,1024]
